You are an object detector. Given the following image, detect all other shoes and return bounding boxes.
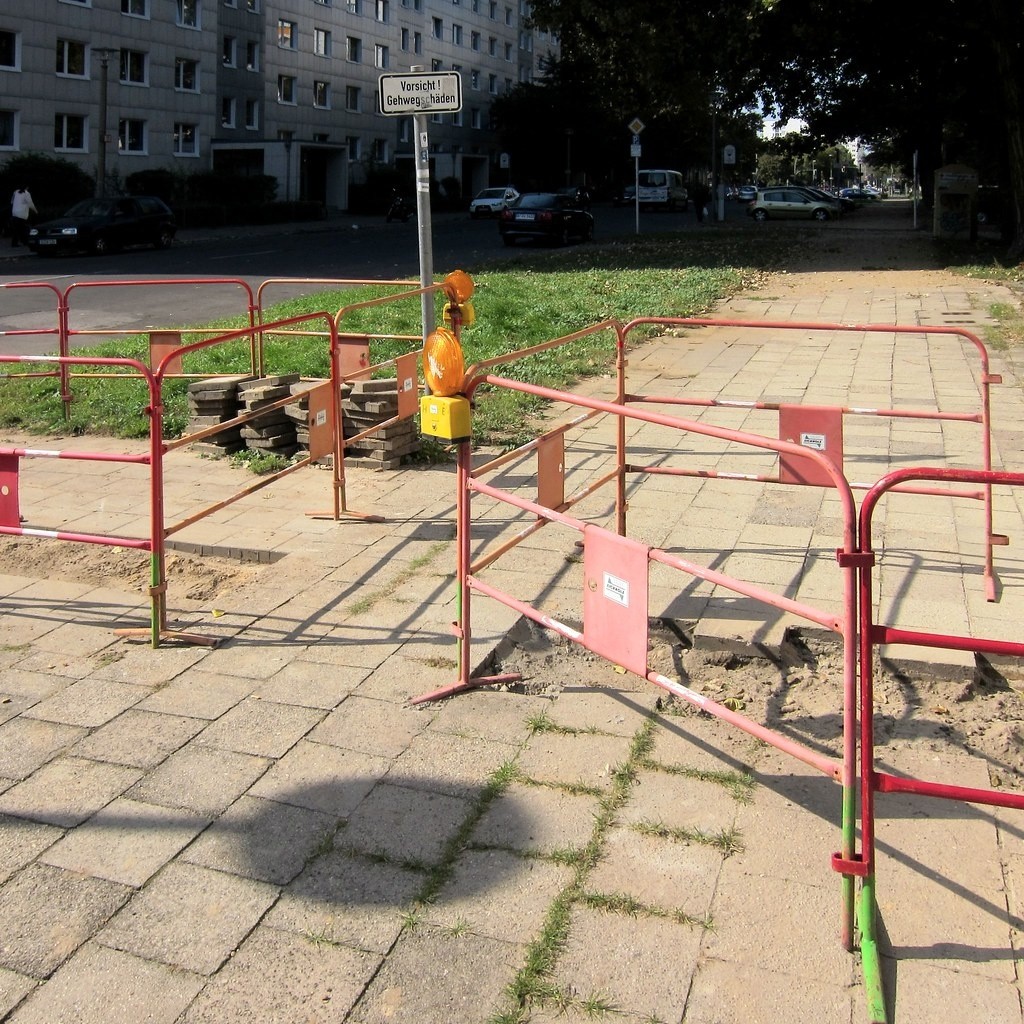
[12,244,21,247]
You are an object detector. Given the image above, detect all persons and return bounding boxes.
[10,179,39,248]
[693,182,706,222]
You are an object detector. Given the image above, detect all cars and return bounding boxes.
[23,195,179,256]
[809,187,856,213]
[834,187,881,202]
[611,185,635,207]
[737,185,757,203]
[470,185,520,214]
[497,192,595,250]
[745,187,842,221]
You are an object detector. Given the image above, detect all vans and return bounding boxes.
[637,170,689,210]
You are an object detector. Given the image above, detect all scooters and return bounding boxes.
[385,187,417,224]
